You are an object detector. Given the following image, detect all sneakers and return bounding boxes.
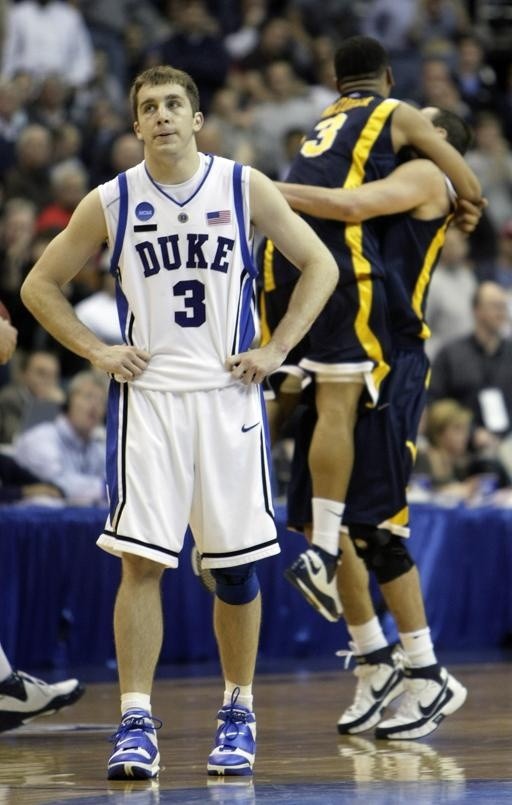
[285,544,345,624]
[107,707,161,782]
[338,644,409,735]
[1,669,85,738]
[375,662,468,741]
[206,706,258,776]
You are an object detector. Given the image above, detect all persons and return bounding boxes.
[0,307,85,739]
[272,101,469,734]
[1,729,79,795]
[0,0,512,513]
[335,733,469,804]
[18,65,346,781]
[258,29,488,630]
[98,776,258,804]
